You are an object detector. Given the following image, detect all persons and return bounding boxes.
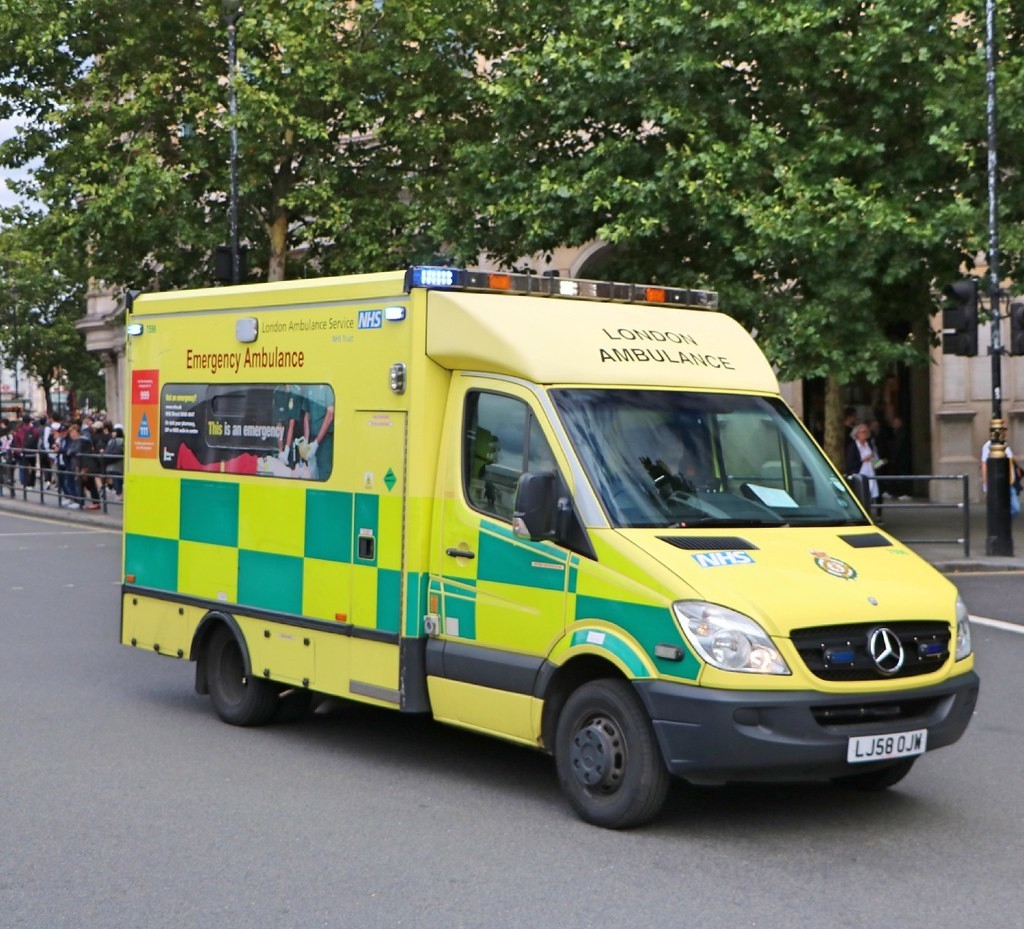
[839,408,914,524]
[638,421,703,498]
[272,382,333,483]
[980,426,1021,518]
[0,405,125,509]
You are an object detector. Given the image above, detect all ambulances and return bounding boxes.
[118,264,983,831]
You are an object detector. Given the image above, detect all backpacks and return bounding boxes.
[20,426,37,449]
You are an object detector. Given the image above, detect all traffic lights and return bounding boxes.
[937,278,981,358]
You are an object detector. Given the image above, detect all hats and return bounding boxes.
[56,425,66,432]
[92,421,104,428]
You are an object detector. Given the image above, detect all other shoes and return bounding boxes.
[875,516,883,525]
[26,486,33,489]
[83,503,100,510]
[61,499,73,506]
[67,502,80,509]
[46,482,51,490]
